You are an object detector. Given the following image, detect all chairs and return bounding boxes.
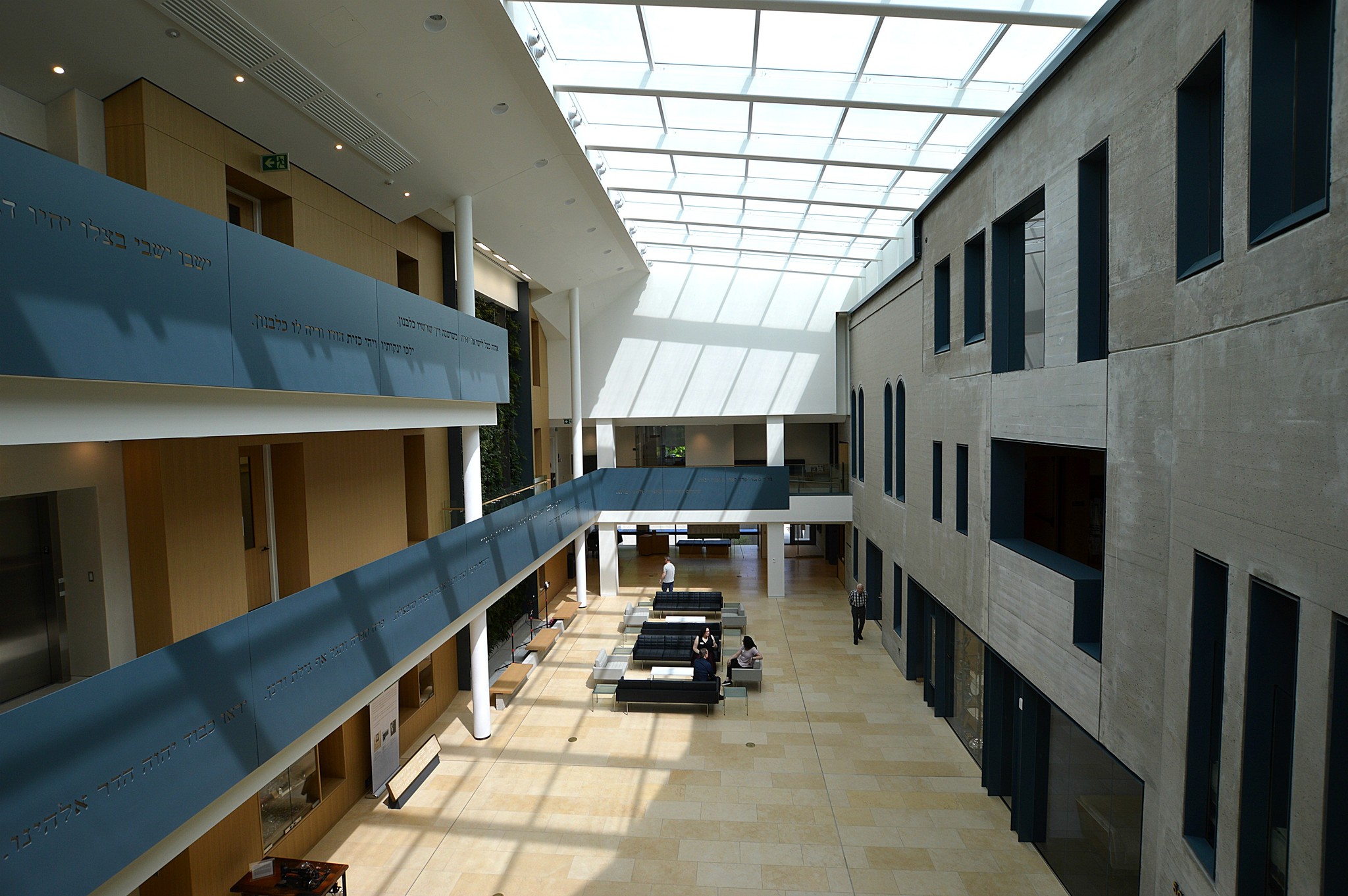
[592,602,649,685]
[721,602,762,693]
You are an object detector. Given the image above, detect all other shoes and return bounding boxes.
[722,681,733,686]
[859,634,863,640]
[721,681,730,686]
[719,694,726,700]
[854,638,858,645]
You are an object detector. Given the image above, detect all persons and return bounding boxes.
[848,584,868,645]
[660,556,675,592]
[691,627,718,674]
[693,648,726,700]
[721,636,763,687]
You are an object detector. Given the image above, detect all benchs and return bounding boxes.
[616,676,721,718]
[525,628,561,662]
[653,592,723,620]
[632,621,723,666]
[489,663,533,710]
[552,602,582,628]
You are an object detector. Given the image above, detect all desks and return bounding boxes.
[592,684,619,712]
[724,687,748,716]
[638,602,653,607]
[665,616,706,623]
[723,629,742,648]
[612,647,634,670]
[230,856,349,896]
[722,649,741,672]
[623,627,642,647]
[724,603,740,608]
[651,667,694,680]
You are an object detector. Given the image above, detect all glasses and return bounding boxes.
[742,640,744,642]
[706,631,710,633]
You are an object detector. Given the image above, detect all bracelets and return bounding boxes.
[752,657,753,660]
[660,579,662,581]
